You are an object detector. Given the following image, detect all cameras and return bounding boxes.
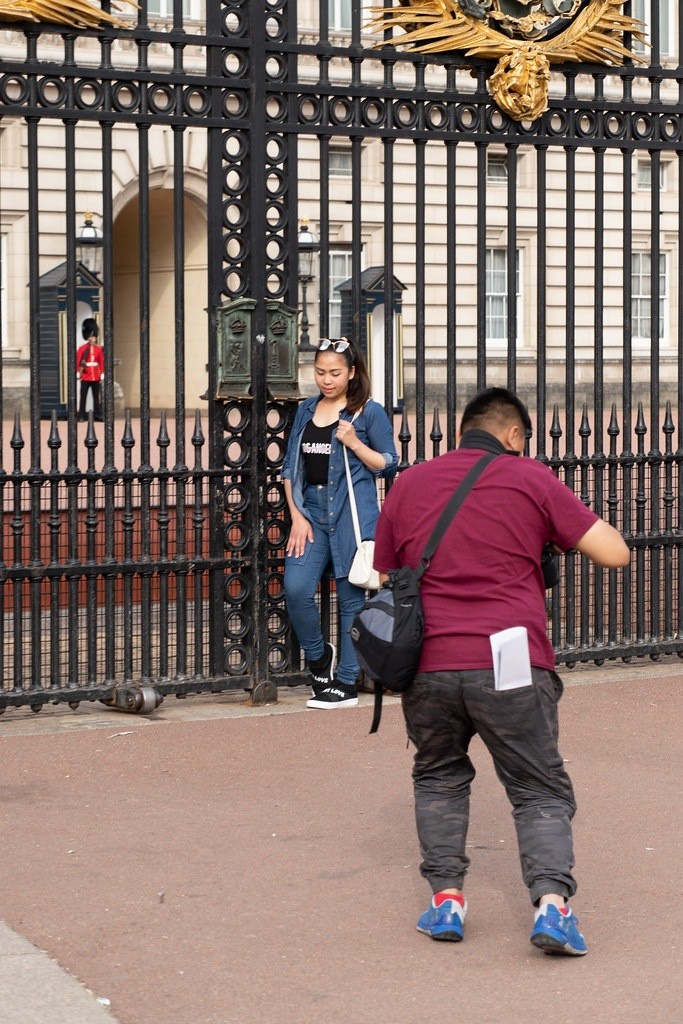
[541,541,560,589]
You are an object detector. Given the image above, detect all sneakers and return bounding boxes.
[416,892,468,942]
[529,903,588,956]
[307,643,336,698]
[307,680,359,709]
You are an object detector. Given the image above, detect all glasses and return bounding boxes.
[318,338,353,356]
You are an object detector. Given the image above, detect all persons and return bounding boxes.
[372,388,630,956]
[76,337,104,421]
[281,338,399,709]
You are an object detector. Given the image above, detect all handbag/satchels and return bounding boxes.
[349,566,426,693]
[542,544,561,589]
[349,540,380,590]
